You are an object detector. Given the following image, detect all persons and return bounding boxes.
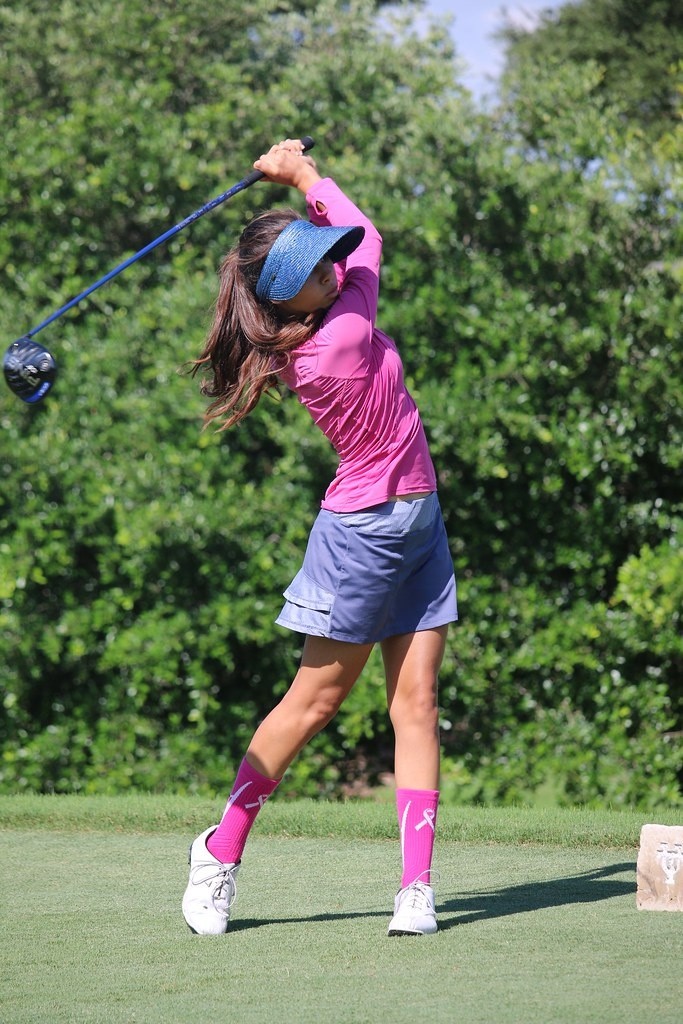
[182,137,459,937]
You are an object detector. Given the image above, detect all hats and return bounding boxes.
[256,219,365,306]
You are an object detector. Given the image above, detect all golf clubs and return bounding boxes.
[4,137,317,401]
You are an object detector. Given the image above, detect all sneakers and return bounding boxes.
[182,825,242,934]
[388,870,440,937]
